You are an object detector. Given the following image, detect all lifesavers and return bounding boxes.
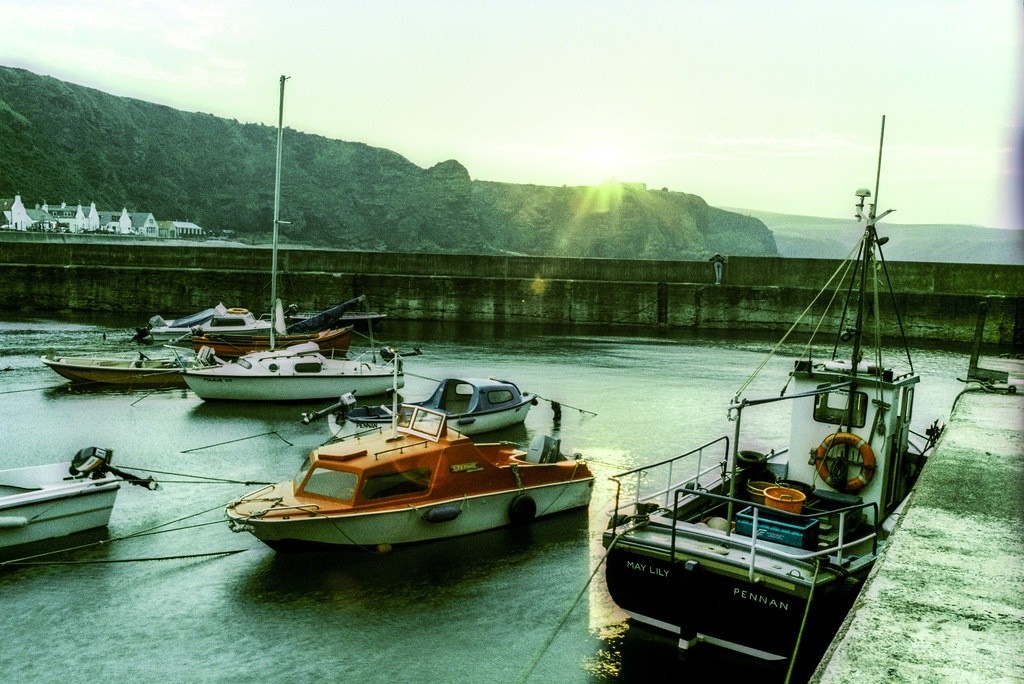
[818,432,874,490]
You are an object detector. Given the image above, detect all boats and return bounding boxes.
[0,448,161,563]
[188,297,356,357]
[300,377,538,445]
[133,301,274,344]
[601,111,944,661]
[259,304,388,330]
[224,348,595,554]
[39,344,234,389]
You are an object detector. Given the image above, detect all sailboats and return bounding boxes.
[178,74,421,402]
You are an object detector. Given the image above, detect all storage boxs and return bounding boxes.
[746,479,776,505]
[735,506,819,552]
[763,483,806,514]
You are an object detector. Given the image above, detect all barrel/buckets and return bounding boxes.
[763,486,806,515]
[747,479,782,505]
